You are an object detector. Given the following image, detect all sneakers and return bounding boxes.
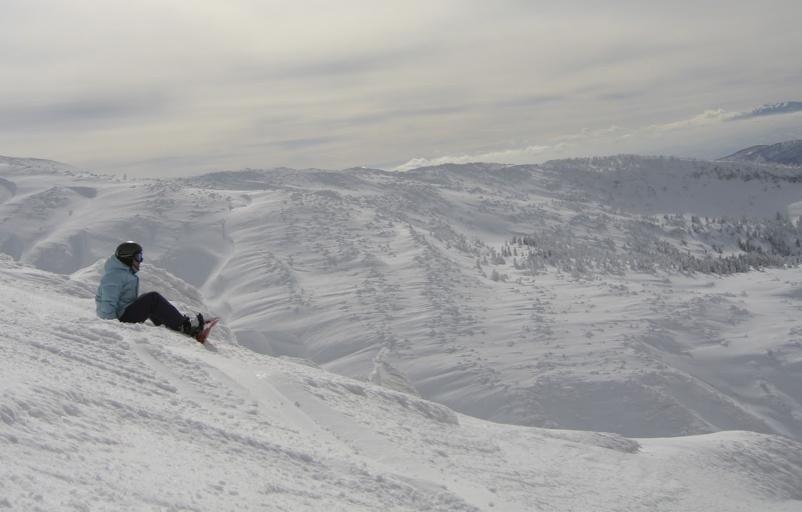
[189,312,215,342]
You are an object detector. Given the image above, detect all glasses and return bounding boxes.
[134,251,143,262]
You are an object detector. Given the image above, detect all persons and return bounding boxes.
[94,241,220,344]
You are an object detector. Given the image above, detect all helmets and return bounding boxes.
[115,241,142,268]
